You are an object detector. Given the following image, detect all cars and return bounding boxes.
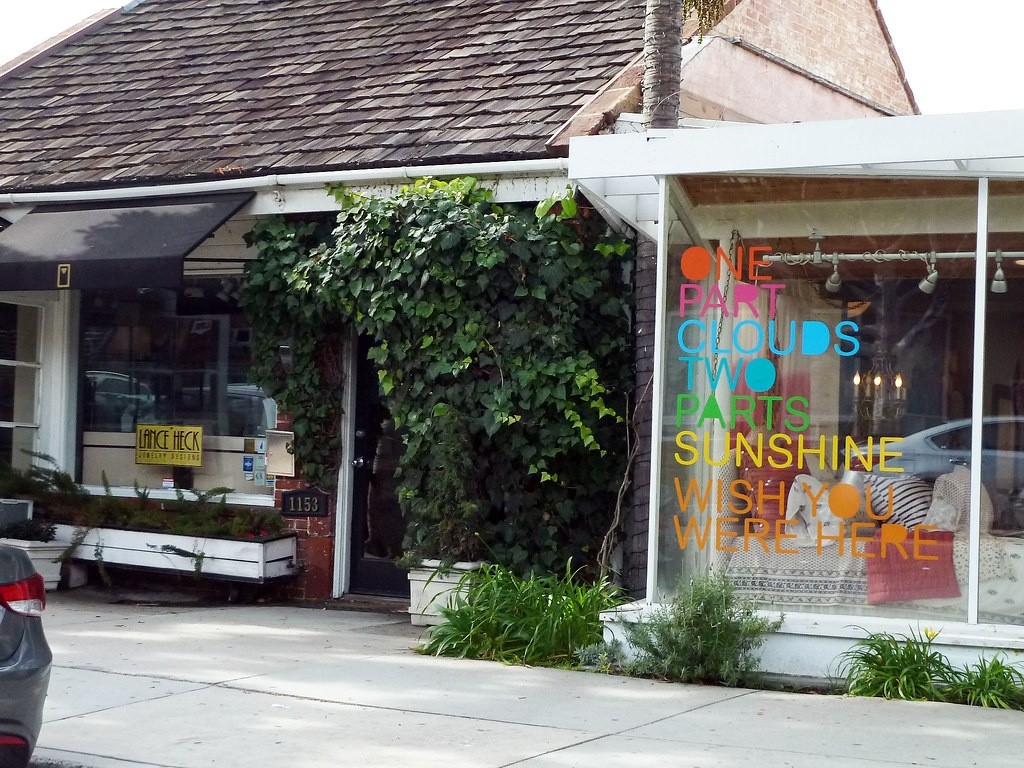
[839,414,1024,529]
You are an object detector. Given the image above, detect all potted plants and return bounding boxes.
[0,517,72,591]
[394,410,493,626]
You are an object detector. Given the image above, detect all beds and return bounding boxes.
[717,425,1024,626]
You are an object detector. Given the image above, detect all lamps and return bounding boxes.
[918,261,941,294]
[990,257,1007,293]
[853,275,907,433]
[825,263,842,292]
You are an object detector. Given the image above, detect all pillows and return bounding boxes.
[863,474,933,530]
[735,447,812,534]
[785,472,857,546]
[932,464,994,532]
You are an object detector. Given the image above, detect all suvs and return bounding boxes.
[88,370,158,421]
[136,384,277,436]
